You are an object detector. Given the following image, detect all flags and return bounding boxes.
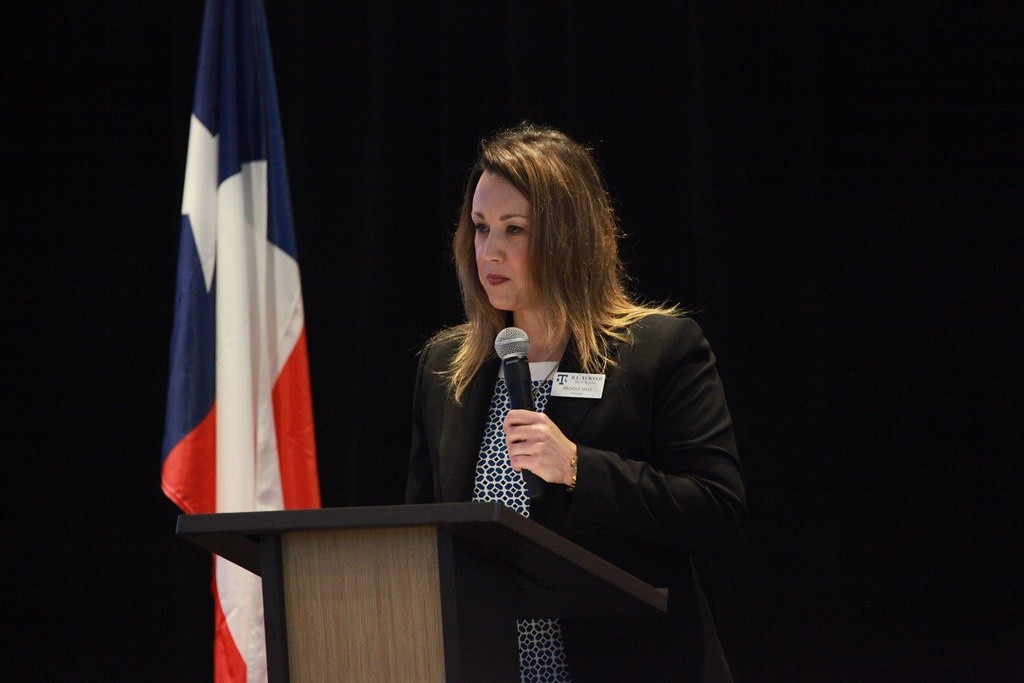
[162,0,320,683]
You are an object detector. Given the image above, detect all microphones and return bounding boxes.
[495,327,540,481]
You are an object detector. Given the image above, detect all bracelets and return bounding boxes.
[566,454,579,493]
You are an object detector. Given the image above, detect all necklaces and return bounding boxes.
[533,361,561,399]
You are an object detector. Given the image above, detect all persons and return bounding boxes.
[406,126,752,683]
